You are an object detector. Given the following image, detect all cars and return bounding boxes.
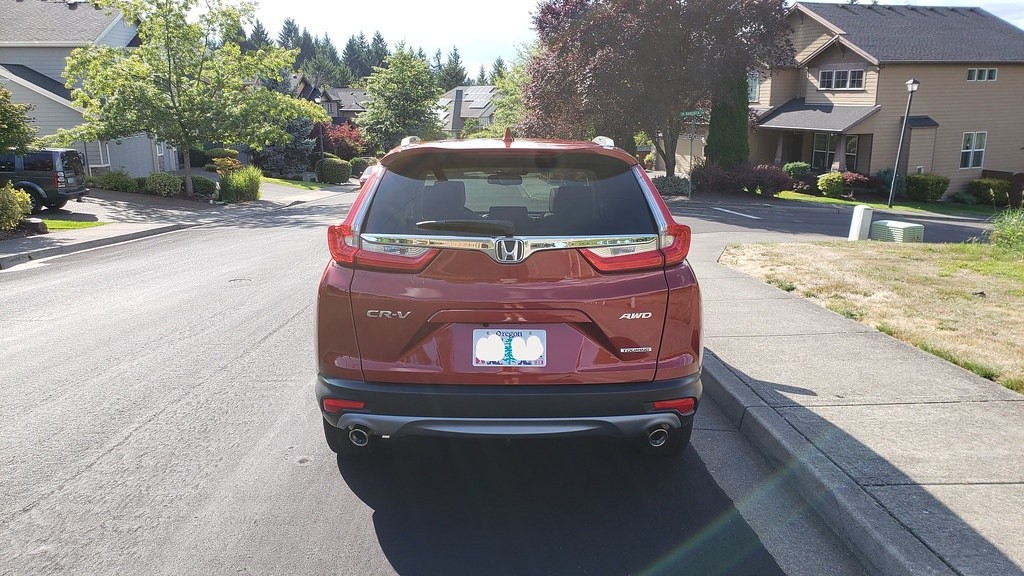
[359,166,375,187]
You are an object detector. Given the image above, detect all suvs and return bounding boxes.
[315,128,703,459]
[0,147,90,214]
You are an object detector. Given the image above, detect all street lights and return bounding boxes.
[887,79,920,208]
